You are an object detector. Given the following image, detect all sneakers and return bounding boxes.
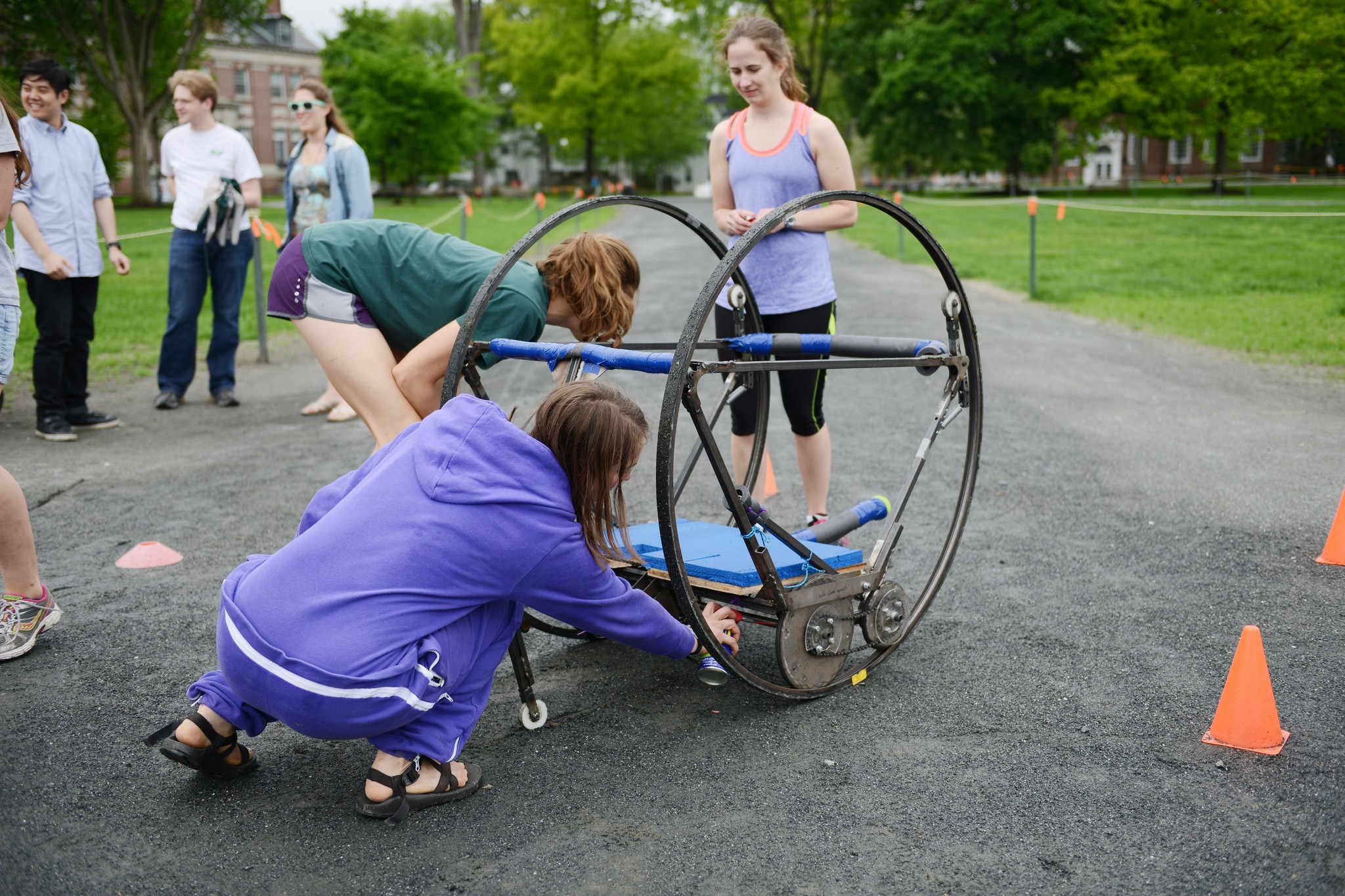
[34,415,79,441]
[154,391,180,408]
[0,583,63,661]
[210,388,239,407]
[71,410,119,431]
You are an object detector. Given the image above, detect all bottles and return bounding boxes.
[698,630,733,685]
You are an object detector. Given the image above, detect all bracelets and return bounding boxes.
[686,624,702,655]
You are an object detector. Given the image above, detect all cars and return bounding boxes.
[148,177,174,204]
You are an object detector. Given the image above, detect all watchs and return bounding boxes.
[785,215,795,229]
[106,241,121,249]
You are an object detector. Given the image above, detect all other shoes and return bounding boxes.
[301,392,342,414]
[329,402,357,420]
[808,516,850,546]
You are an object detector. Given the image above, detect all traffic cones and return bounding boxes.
[1313,487,1345,566]
[1201,626,1289,756]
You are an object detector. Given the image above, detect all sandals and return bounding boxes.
[142,710,256,774]
[355,755,481,825]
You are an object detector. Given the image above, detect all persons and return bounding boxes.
[154,69,262,409]
[9,56,130,441]
[0,96,65,661]
[267,217,641,458]
[278,78,375,421]
[144,378,739,827]
[708,16,857,551]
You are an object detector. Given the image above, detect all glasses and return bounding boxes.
[288,98,327,113]
